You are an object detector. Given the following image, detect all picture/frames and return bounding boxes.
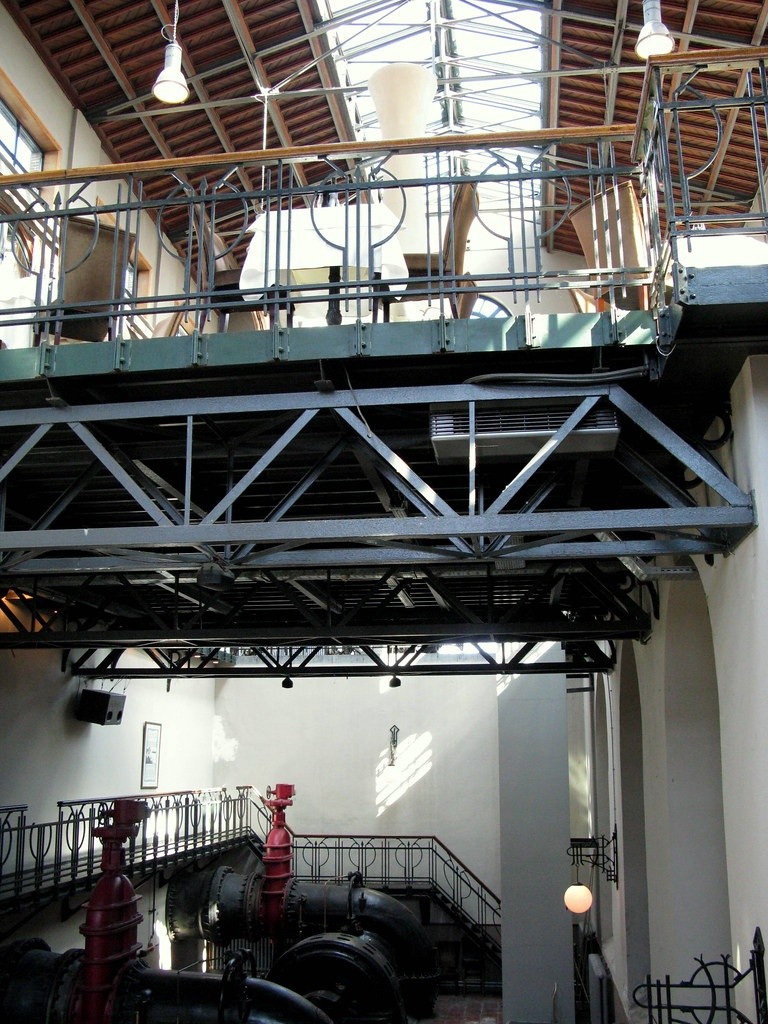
[140,722,162,789]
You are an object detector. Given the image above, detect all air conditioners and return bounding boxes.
[431,405,620,472]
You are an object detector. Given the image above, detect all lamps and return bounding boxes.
[151,0,190,104]
[635,0,675,60]
[390,645,400,687]
[564,846,593,913]
[282,676,293,688]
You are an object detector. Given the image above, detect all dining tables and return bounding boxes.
[239,204,410,329]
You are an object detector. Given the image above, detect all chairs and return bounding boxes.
[568,180,674,312]
[453,272,478,319]
[167,203,294,337]
[33,217,136,347]
[569,289,611,313]
[373,183,479,319]
